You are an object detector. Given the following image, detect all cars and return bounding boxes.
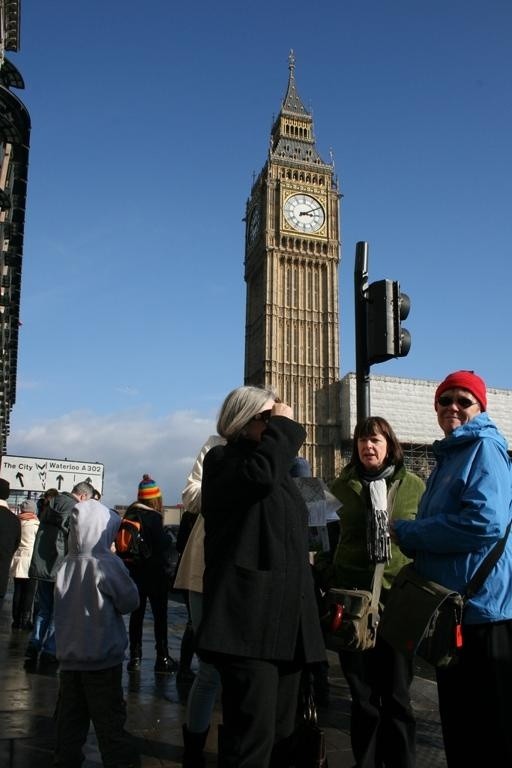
[164,523,180,587]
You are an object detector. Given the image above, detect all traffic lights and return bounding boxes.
[367,275,412,365]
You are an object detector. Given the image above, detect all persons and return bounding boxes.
[1,478,101,675]
[53,499,140,767]
[175,437,228,766]
[387,369,512,768]
[289,457,327,551]
[111,474,196,683]
[313,415,428,767]
[198,384,326,767]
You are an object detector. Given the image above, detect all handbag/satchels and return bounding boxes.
[375,564,464,681]
[297,672,328,767]
[320,586,384,653]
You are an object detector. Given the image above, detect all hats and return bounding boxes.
[435,371,487,411]
[20,499,36,512]
[1,479,10,499]
[137,474,161,500]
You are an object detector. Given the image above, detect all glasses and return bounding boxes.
[253,409,272,428]
[438,396,473,408]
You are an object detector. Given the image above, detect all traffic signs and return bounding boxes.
[1,454,104,499]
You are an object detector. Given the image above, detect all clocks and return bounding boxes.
[283,192,327,234]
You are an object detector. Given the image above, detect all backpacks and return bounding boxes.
[114,508,145,552]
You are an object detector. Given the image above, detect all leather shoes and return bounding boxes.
[153,656,178,675]
[127,658,141,671]
